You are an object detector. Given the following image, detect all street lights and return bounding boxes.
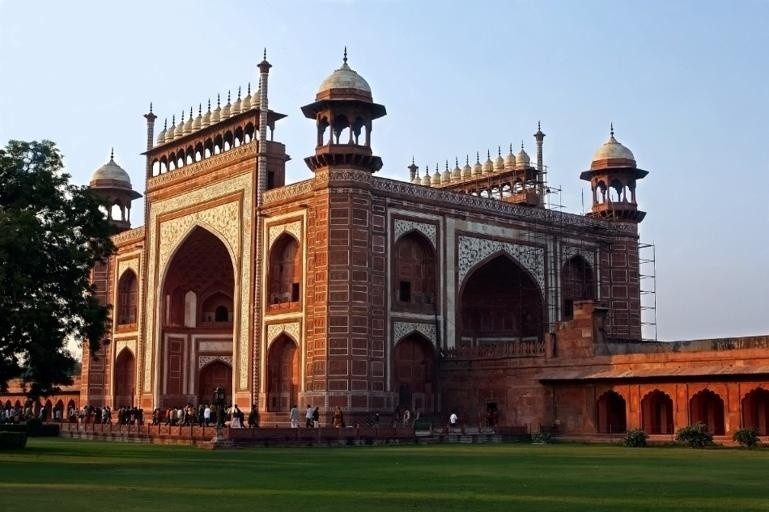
[213,386,225,426]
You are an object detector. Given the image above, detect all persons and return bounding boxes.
[1,405,144,425]
[152,403,260,429]
[368,405,422,428]
[335,407,346,428]
[449,410,460,433]
[289,404,319,429]
